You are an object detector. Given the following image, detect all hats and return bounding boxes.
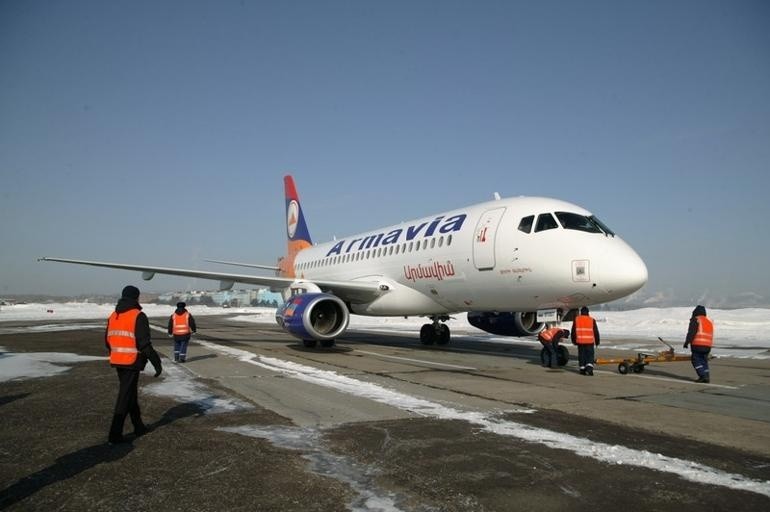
[122,285,140,299]
[563,329,570,339]
[177,302,186,308]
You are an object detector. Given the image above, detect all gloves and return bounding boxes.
[153,363,163,377]
[683,341,689,349]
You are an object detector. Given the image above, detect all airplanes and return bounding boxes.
[36,173,650,371]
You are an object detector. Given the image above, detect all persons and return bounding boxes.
[682,304,714,383]
[538,327,570,370]
[571,306,600,376]
[167,302,197,362]
[104,285,162,446]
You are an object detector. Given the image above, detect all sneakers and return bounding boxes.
[695,376,710,383]
[134,424,153,436]
[580,366,594,376]
[543,364,560,369]
[108,433,124,447]
[174,356,185,363]
